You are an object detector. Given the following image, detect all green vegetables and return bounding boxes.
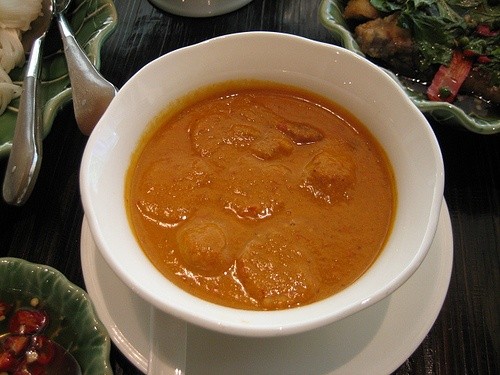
[369,0,500,99]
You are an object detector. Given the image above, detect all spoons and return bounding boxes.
[52,0,120,137]
[3,0,53,207]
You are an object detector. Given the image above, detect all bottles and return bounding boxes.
[80,195,453,375]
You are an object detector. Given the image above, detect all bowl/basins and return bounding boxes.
[79,31,444,340]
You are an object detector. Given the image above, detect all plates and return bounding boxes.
[0,257,113,375]
[0,0,118,161]
[319,0,500,135]
